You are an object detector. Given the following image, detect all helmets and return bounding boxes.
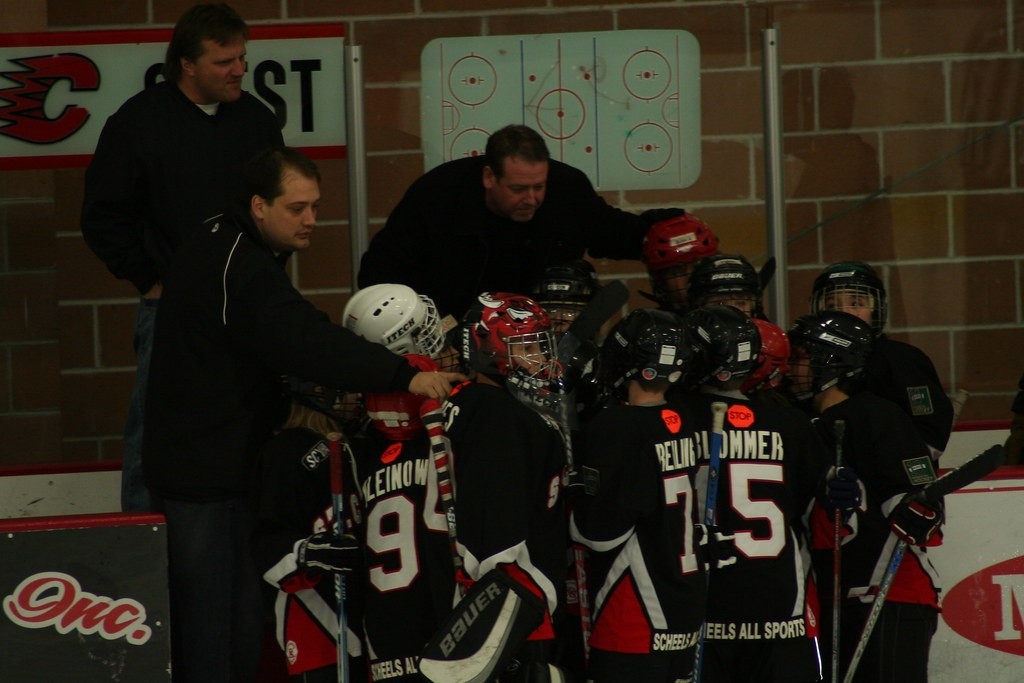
[343,284,446,358]
[788,312,875,399]
[743,317,790,395]
[535,259,598,325]
[689,255,767,315]
[642,214,722,294]
[508,331,601,420]
[685,307,761,389]
[366,354,442,440]
[601,311,691,395]
[814,262,889,335]
[457,290,557,383]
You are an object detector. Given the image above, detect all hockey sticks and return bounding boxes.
[418,397,468,600]
[833,419,845,683]
[754,252,777,319]
[326,430,351,683]
[555,278,630,675]
[687,401,729,683]
[843,443,1010,683]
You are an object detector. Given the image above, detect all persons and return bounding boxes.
[1005,375,1024,465]
[259,126,955,683]
[143,146,468,682]
[80,1,285,513]
